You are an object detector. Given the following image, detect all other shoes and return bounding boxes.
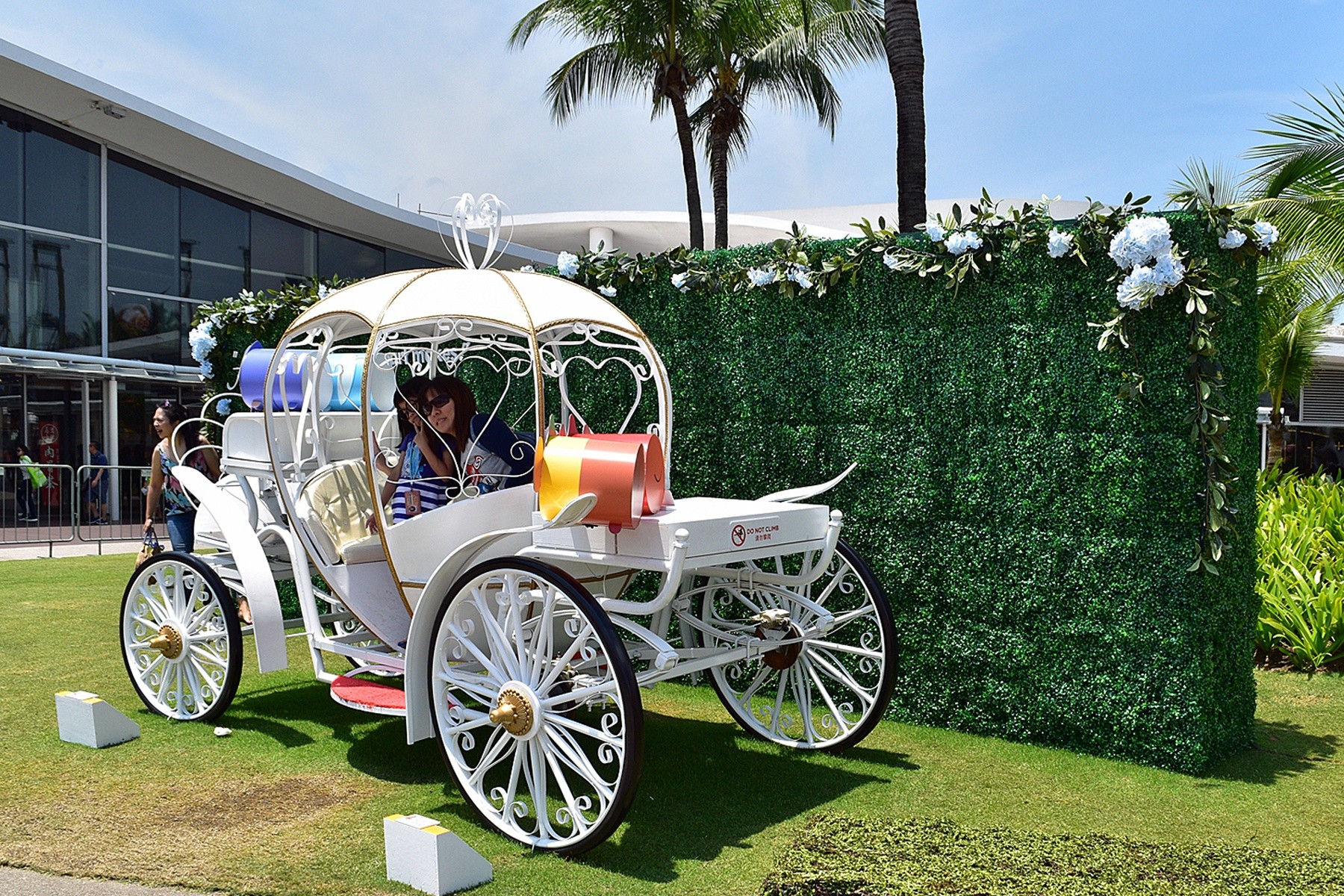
[24,516,38,522]
[18,514,31,521]
[96,520,108,524]
[90,516,103,523]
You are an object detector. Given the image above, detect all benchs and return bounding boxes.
[294,456,399,566]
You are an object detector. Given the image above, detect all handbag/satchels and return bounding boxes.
[470,413,536,456]
[27,463,47,488]
[81,478,89,491]
[135,529,164,570]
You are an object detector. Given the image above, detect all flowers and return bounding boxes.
[515,189,1281,575]
[188,274,366,445]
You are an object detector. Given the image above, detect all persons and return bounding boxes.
[10,446,49,521]
[82,441,110,525]
[135,400,223,611]
[365,374,537,533]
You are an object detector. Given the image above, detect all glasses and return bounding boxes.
[89,444,94,447]
[160,400,181,419]
[398,399,419,421]
[423,394,451,416]
[16,449,22,452]
